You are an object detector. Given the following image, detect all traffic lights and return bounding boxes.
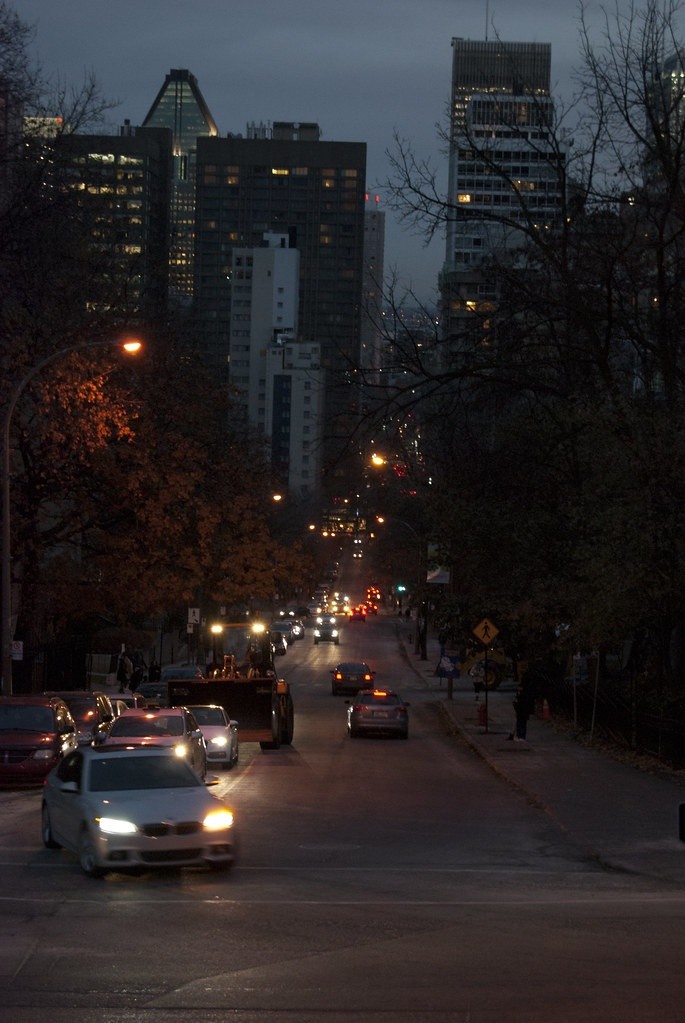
[398,586,405,592]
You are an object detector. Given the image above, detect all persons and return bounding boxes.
[470,661,485,701]
[405,608,410,623]
[117,648,159,694]
[507,684,530,741]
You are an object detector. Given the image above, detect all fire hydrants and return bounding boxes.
[477,704,487,725]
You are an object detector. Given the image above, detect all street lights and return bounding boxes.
[2,337,143,695]
[370,450,429,660]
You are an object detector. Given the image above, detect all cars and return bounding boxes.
[184,705,239,770]
[344,689,411,739]
[102,705,207,780]
[270,546,381,657]
[0,666,207,782]
[329,662,377,696]
[42,743,238,880]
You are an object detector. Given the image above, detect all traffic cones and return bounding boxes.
[543,699,551,719]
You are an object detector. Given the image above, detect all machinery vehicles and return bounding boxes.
[167,623,294,750]
